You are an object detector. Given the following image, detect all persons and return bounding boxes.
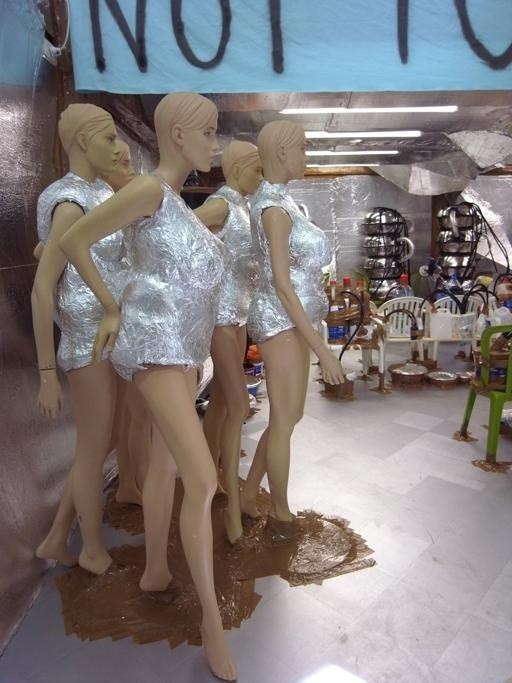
[22,97,137,578]
[191,135,261,540]
[31,138,152,508]
[239,114,348,526]
[59,90,243,683]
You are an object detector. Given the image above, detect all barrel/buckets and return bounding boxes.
[341,274,363,344]
[497,277,512,312]
[351,279,373,345]
[245,344,268,394]
[324,280,349,343]
[393,274,415,299]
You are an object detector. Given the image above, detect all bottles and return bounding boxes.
[325,276,371,340]
[394,273,416,298]
[446,272,466,295]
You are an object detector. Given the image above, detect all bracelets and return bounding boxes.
[36,360,58,371]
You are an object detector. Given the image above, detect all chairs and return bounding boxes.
[320,294,512,462]
